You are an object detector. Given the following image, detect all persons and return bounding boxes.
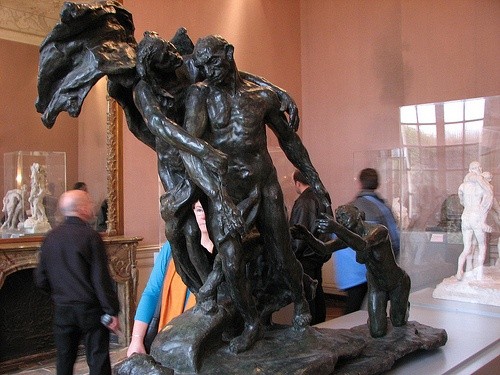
[333,168,401,316]
[35,190,121,375]
[0,162,53,232]
[290,203,411,337]
[133,35,335,352]
[455,162,500,284]
[289,169,327,324]
[74,181,88,193]
[127,200,214,357]
